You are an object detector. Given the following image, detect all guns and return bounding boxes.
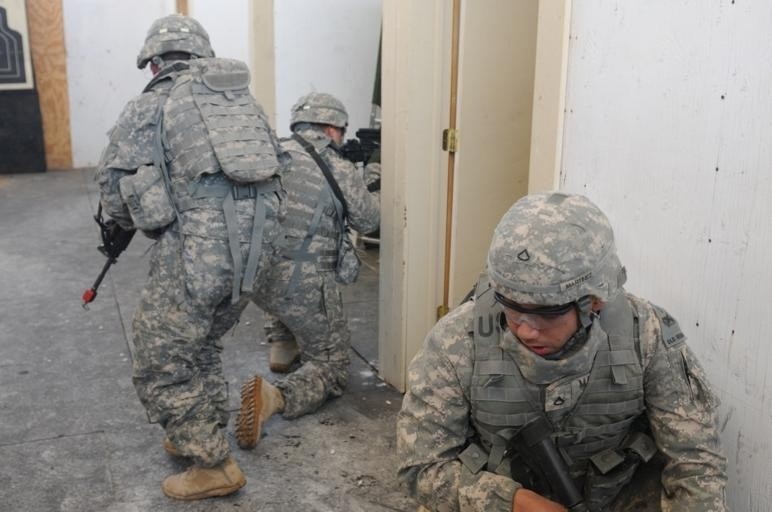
[81,199,136,302]
[502,419,584,510]
[339,129,380,169]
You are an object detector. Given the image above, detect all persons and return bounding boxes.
[92,12,287,500]
[393,192,729,511]
[235,90,381,450]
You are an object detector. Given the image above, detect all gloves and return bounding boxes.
[105,220,137,249]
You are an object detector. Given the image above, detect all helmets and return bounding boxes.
[290,91,348,131]
[137,14,215,70]
[485,193,624,306]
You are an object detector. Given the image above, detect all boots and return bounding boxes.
[235,376,284,450]
[270,338,299,371]
[161,437,247,500]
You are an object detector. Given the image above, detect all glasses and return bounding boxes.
[493,291,574,331]
